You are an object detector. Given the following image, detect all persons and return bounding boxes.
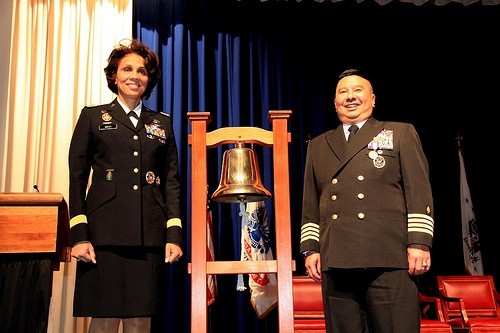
[67,39,186,333]
[301,70,435,333]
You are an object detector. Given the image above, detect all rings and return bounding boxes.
[177,259,178,261]
[305,270,309,275]
[77,259,80,261]
[422,262,427,267]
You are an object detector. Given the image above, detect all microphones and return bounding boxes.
[33,185,39,193]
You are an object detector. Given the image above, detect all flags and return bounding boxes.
[241,200,278,319]
[206,182,219,306]
[457,151,483,276]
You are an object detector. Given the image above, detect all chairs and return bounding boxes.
[420,276,500,333]
[292,276,326,333]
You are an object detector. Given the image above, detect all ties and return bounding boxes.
[348,124,359,142]
